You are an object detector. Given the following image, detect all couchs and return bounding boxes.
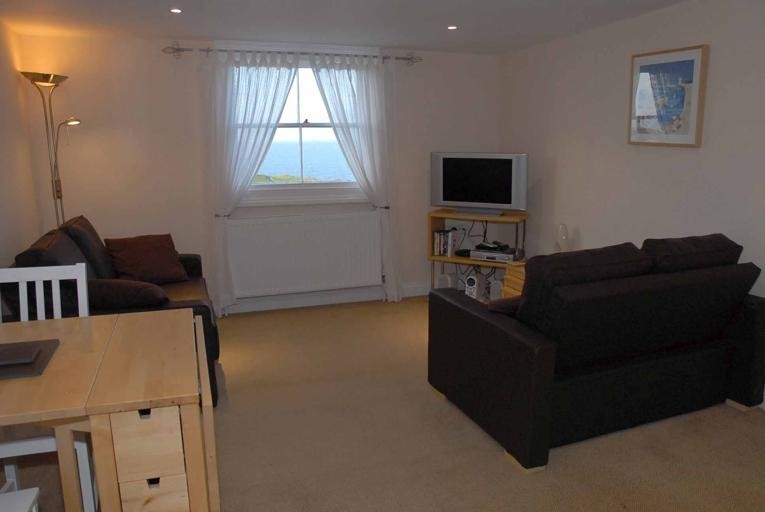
[0,215,220,407]
[427,262,765,470]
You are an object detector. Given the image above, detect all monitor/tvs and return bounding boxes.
[430,150,530,217]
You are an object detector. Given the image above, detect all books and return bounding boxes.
[433,228,459,258]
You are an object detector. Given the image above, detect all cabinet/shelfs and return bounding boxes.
[426,209,529,291]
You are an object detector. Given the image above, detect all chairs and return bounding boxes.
[0,263,100,512]
[0,487,41,512]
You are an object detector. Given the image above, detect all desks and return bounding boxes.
[0,309,221,512]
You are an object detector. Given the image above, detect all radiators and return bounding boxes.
[224,212,383,299]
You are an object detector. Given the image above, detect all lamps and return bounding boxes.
[19,71,82,226]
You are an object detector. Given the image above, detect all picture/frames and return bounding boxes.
[627,42,709,148]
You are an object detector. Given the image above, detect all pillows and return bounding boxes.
[640,233,744,272]
[522,242,652,297]
[88,279,170,312]
[103,233,188,285]
